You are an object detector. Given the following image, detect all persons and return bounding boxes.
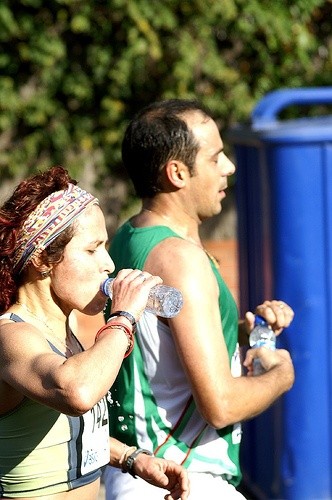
[104,100,296,500]
[0,168,190,500]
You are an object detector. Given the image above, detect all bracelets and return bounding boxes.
[126,448,151,479]
[106,311,136,335]
[144,208,220,268]
[94,323,134,358]
[118,446,136,473]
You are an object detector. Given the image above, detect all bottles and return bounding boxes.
[249,315,277,375]
[99,277,184,319]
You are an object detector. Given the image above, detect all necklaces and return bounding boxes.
[24,304,80,355]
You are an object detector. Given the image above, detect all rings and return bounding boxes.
[280,305,284,308]
